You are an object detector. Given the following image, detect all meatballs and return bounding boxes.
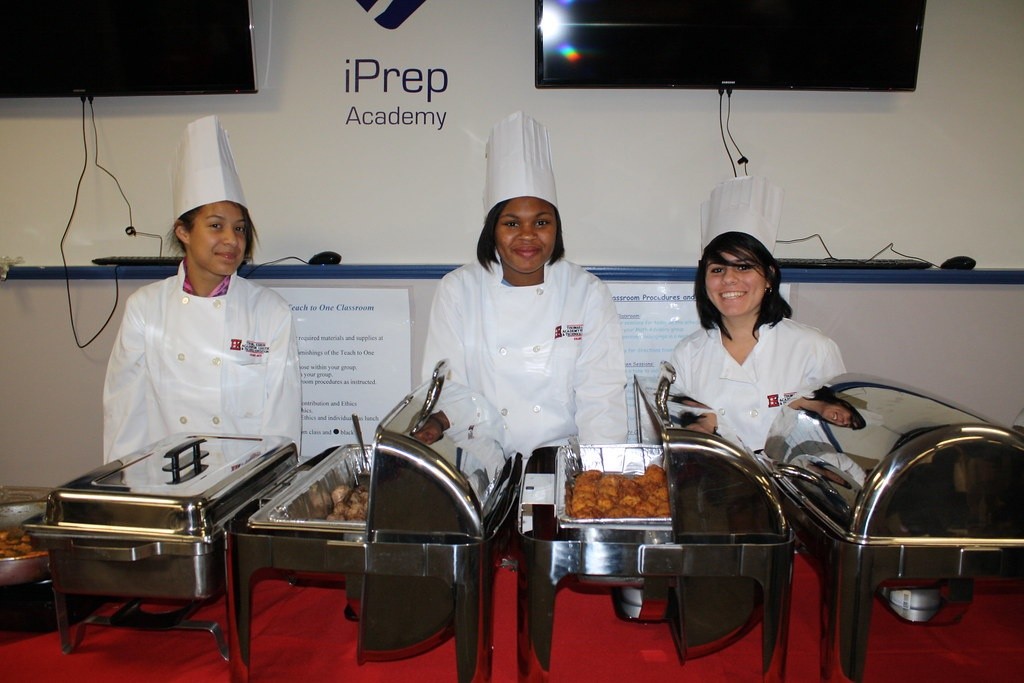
[326,485,367,520]
[571,464,671,519]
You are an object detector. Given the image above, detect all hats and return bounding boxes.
[701,176,785,255]
[175,115,248,222]
[484,110,558,216]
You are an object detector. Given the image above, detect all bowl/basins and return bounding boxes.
[0,501,48,585]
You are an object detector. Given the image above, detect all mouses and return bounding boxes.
[941,256,979,271]
[309,251,341,266]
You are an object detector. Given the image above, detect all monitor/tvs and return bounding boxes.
[534,0,927,93]
[0,0,259,99]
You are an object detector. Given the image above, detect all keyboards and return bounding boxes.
[777,256,932,270]
[93,255,188,268]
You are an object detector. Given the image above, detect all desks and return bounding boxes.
[0,553,1024,683]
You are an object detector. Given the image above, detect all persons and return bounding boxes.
[101,183,303,466]
[421,181,630,483]
[670,395,748,456]
[765,385,868,462]
[409,377,506,482]
[667,216,849,457]
